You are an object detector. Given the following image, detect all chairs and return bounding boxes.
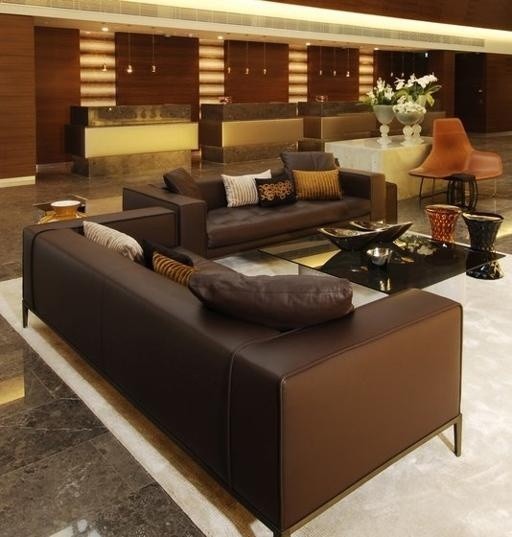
[407,114,504,206]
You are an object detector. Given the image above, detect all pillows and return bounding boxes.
[292,167,348,205]
[278,148,337,173]
[146,242,193,270]
[159,163,205,202]
[80,219,142,263]
[220,167,273,208]
[187,261,356,323]
[254,173,297,206]
[146,250,197,285]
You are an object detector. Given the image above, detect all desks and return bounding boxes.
[31,193,86,215]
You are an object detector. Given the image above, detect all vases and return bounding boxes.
[412,114,424,144]
[371,104,396,145]
[37,200,88,224]
[395,112,424,145]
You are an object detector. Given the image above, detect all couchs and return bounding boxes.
[121,156,399,259]
[20,204,467,536]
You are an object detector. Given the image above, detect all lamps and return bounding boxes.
[100,28,351,78]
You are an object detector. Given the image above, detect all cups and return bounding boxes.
[427,242,501,281]
[371,267,391,291]
[460,212,505,252]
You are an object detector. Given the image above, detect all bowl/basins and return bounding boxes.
[323,250,369,274]
[366,248,393,266]
[313,221,412,247]
[49,200,82,217]
[373,243,417,264]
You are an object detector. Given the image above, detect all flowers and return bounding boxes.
[366,72,442,114]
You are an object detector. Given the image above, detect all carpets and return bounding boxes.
[0,228,511,537]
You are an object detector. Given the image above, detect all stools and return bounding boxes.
[447,173,479,211]
[460,211,503,280]
